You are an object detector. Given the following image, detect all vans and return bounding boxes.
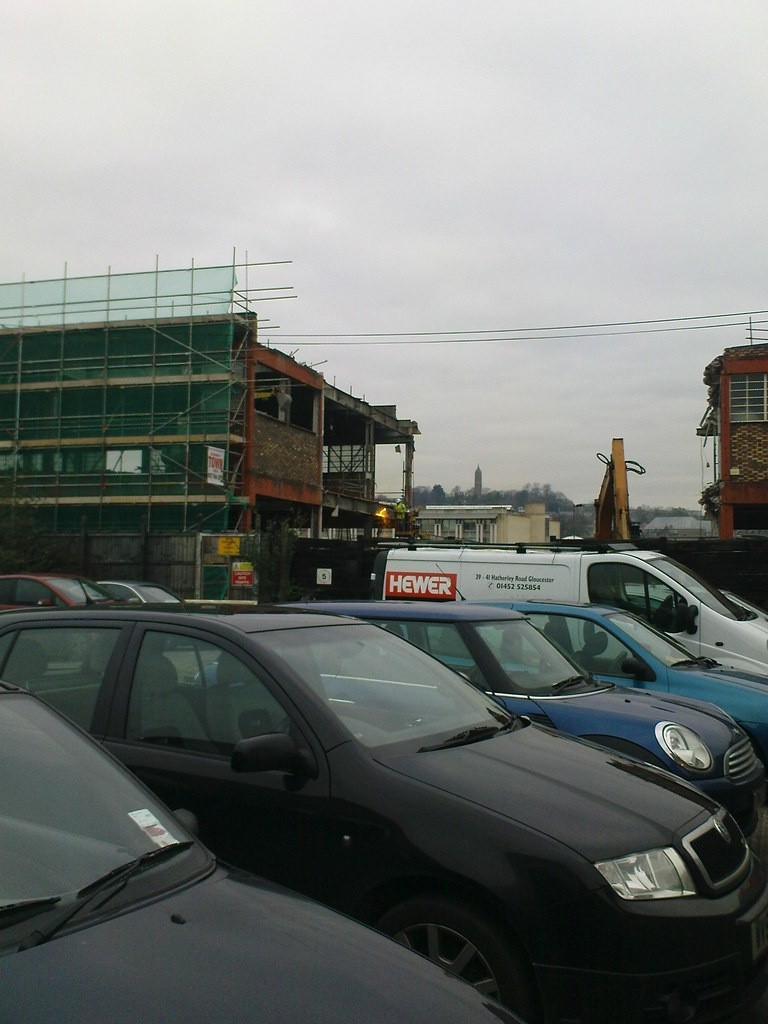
[377,530,768,683]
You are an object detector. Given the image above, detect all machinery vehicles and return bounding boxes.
[592,437,646,543]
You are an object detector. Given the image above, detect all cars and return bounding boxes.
[0,572,137,615]
[0,598,768,1024]
[55,580,187,607]
[1,677,528,1024]
[382,599,767,778]
[196,597,767,840]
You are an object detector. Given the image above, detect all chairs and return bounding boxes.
[544,622,572,655]
[203,651,296,744]
[141,652,209,742]
[384,623,405,638]
[499,629,525,665]
[438,629,464,653]
